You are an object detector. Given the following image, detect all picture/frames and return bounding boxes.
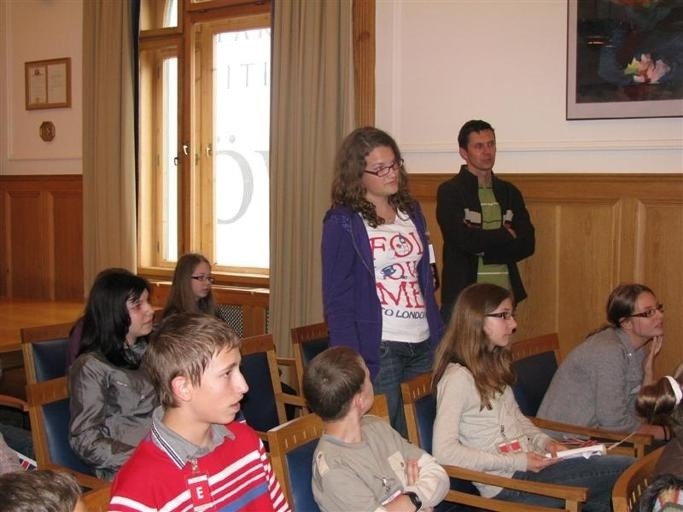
[24,57,71,110]
[565,0,683,121]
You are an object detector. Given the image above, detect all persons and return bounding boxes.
[436,121,535,325]
[535,284,683,512]
[68,253,221,482]
[1,470,86,512]
[303,346,450,511]
[106,313,292,512]
[430,281,638,509]
[321,127,448,438]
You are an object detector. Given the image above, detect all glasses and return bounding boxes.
[363,157,405,177]
[626,304,664,318]
[484,312,516,320]
[191,275,215,283]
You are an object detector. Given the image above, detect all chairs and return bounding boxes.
[401,370,591,511]
[266,393,571,511]
[611,438,683,510]
[19,320,81,393]
[238,332,300,454]
[506,331,654,458]
[0,393,38,471]
[289,319,331,384]
[276,356,301,397]
[26,369,109,492]
[80,484,111,511]
